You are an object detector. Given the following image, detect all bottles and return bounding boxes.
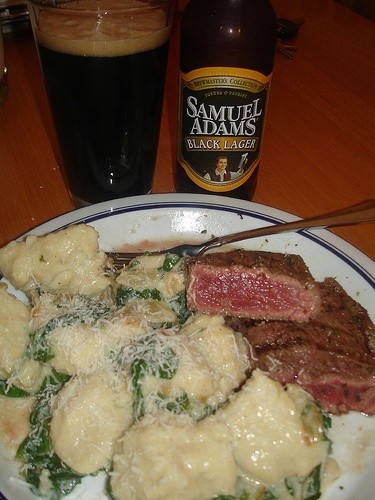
[175,0,279,203]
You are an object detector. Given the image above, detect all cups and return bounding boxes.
[26,1,175,211]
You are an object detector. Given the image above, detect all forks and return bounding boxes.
[106,195,375,269]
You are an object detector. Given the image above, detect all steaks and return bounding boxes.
[186,248,375,417]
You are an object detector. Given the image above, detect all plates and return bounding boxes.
[1,193,375,499]
